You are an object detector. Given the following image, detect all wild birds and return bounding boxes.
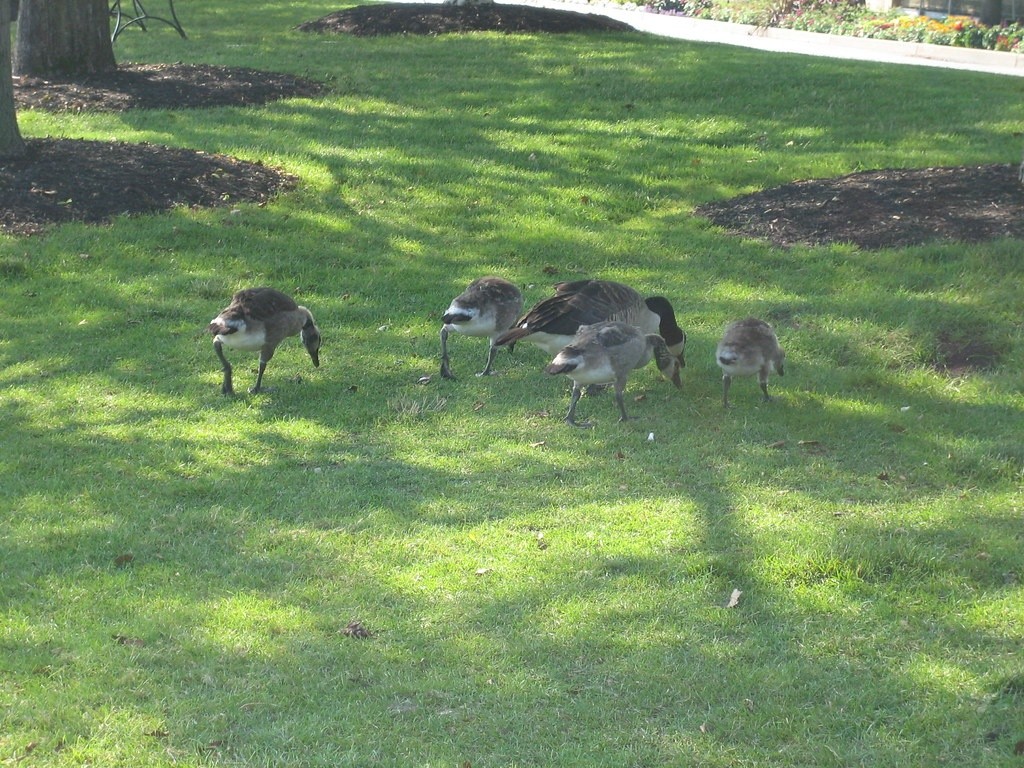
[492,280,686,393]
[545,321,681,429]
[715,318,785,407]
[208,288,321,397]
[439,276,522,380]
